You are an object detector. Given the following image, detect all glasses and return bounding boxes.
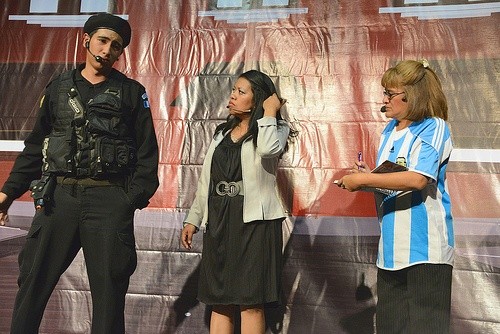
[383,91,405,99]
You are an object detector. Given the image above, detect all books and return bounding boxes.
[333,160,408,195]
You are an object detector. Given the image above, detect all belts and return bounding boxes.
[40,175,125,186]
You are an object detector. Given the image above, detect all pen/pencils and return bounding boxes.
[358,151,363,171]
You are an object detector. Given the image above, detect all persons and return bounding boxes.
[336,58,455,334]
[0,13,160,334]
[180,70,300,334]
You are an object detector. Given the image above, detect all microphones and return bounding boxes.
[381,106,386,112]
[227,106,252,113]
[85,42,102,62]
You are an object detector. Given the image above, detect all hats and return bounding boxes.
[83,13,131,49]
